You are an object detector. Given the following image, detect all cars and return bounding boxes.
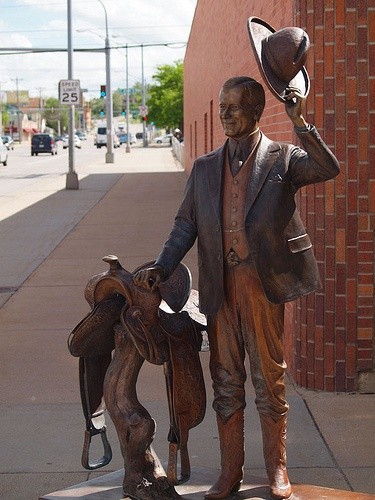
[0,137,9,167]
[153,134,173,144]
[119,133,132,145]
[63,135,81,149]
[1,135,14,150]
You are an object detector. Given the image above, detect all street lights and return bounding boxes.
[111,34,148,148]
[77,29,131,153]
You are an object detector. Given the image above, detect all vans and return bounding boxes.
[32,133,58,155]
[95,126,120,148]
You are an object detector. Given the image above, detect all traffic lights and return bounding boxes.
[100,109,105,115]
[101,85,106,97]
[121,112,126,116]
[150,121,154,125]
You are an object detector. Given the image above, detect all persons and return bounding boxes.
[133,76,339,500]
